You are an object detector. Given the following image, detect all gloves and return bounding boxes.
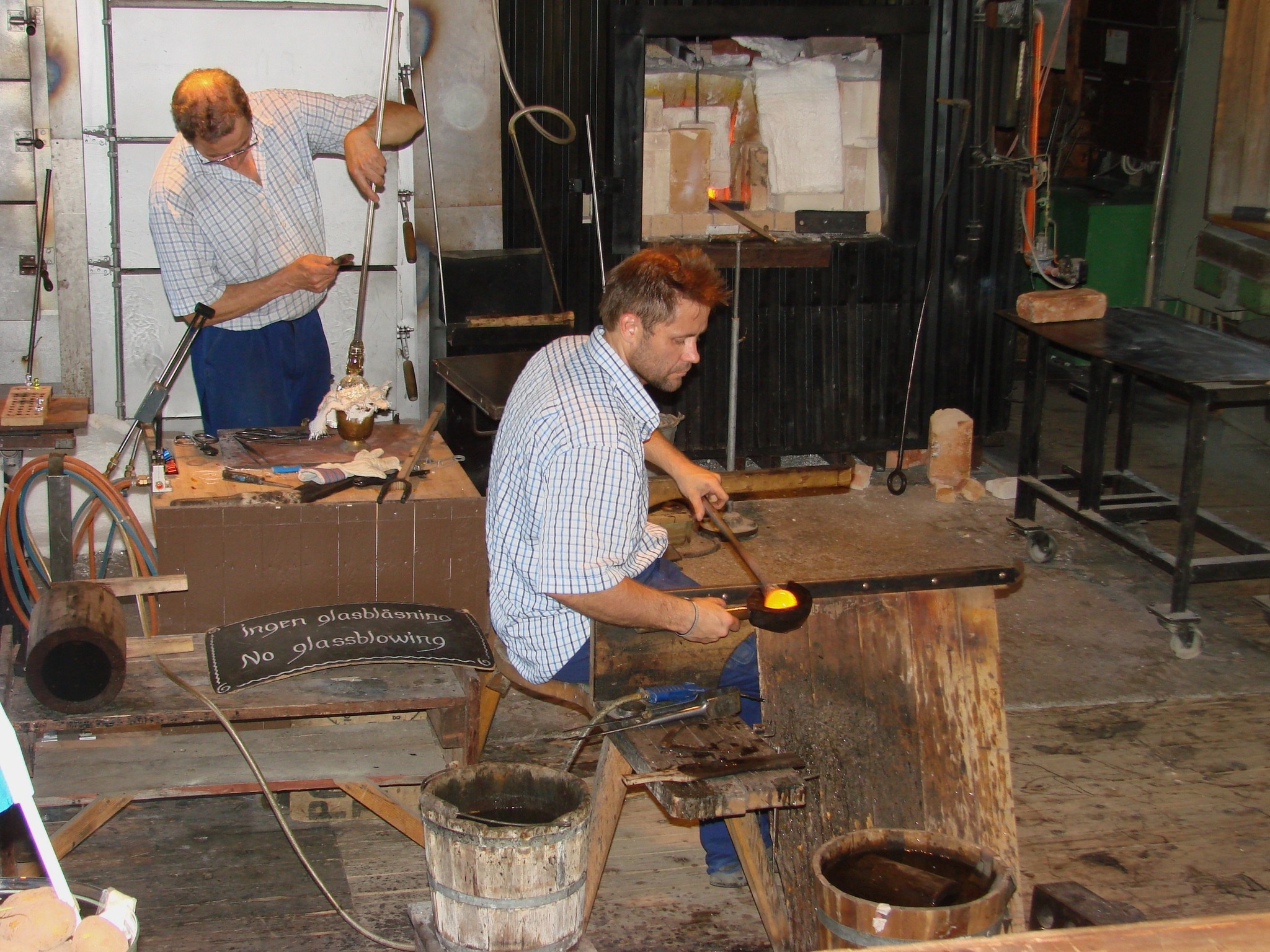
[298,448,402,486]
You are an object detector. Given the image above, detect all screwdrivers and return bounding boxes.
[222,470,293,488]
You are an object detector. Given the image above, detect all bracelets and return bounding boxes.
[676,597,698,637]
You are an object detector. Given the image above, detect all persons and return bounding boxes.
[149,69,422,438]
[485,242,777,888]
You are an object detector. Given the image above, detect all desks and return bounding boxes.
[997,305,1270,658]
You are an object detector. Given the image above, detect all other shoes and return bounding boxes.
[709,845,780,887]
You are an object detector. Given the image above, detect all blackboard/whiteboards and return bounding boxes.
[204,601,497,695]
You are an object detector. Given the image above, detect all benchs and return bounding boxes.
[477,653,806,952]
[33,718,447,858]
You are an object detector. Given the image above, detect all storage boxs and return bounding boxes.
[290,711,430,821]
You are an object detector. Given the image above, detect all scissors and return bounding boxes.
[235,428,296,440]
[175,433,218,448]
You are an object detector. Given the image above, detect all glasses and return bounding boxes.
[193,124,259,167]
